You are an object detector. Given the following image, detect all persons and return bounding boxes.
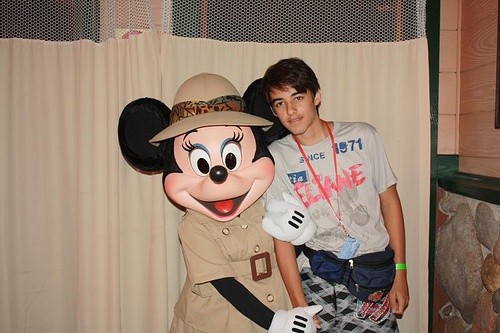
[265,58,410,333]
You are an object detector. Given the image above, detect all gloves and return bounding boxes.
[267,303,323,333]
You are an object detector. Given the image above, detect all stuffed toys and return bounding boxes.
[118,73,323,333]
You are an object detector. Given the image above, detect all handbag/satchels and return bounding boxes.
[303,244,396,303]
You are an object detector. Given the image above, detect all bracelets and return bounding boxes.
[395,262,407,270]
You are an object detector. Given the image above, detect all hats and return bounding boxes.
[148,72,274,142]
